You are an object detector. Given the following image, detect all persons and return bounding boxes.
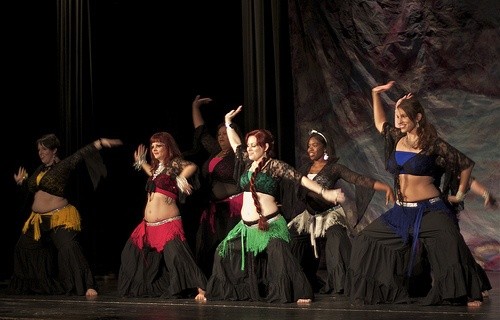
[7,134,123,296]
[117,81,498,308]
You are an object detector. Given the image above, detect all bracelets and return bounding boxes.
[135,157,148,169]
[98,137,104,149]
[481,188,490,201]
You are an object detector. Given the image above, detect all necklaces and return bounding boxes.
[403,135,420,150]
